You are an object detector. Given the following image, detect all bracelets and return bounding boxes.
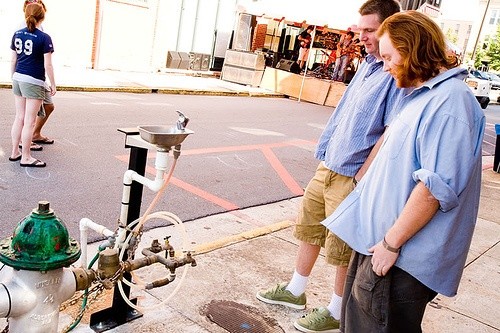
[383,236,400,253]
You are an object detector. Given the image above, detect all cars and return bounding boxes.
[480,72,500,104]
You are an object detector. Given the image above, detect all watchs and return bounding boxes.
[353,176,359,187]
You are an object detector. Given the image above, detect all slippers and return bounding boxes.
[19,141,43,150]
[9,151,22,160]
[19,156,46,167]
[32,136,54,144]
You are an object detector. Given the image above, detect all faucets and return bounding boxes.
[163,235,175,258]
[182,248,197,267]
[176,110,188,130]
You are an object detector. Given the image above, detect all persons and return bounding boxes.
[256,0,407,333]
[319,9,485,333]
[331,31,354,81]
[17,0,54,151]
[297,25,314,69]
[9,2,56,168]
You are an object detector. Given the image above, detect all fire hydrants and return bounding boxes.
[0,201,97,333]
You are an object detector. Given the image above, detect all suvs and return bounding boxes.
[465,69,491,109]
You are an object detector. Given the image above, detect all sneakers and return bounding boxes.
[256,282,307,309]
[293,305,341,332]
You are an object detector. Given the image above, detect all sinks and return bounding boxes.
[138,125,195,146]
